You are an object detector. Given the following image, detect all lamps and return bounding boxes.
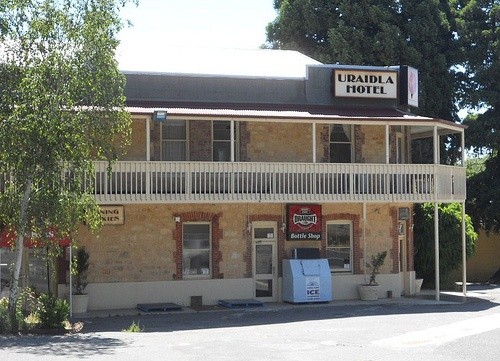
[153,111,167,121]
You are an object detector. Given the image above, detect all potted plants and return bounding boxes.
[413,246,423,293]
[356,250,388,301]
[67,244,91,312]
